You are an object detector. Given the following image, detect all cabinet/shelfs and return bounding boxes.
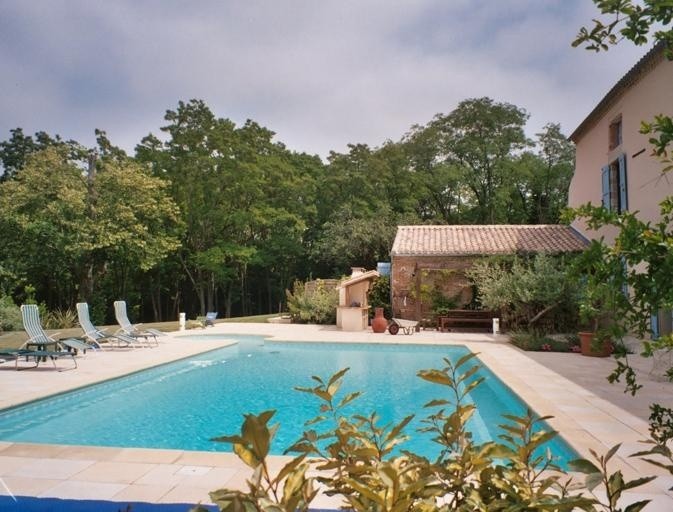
[340,307,368,330]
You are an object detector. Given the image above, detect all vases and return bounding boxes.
[371,307,387,332]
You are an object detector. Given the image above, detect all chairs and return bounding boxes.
[53,334,96,360]
[114,300,160,350]
[73,298,128,351]
[0,344,73,372]
[17,303,80,369]
[197,311,217,328]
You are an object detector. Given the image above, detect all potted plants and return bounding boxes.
[569,249,612,358]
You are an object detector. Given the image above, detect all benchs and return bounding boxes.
[436,308,503,333]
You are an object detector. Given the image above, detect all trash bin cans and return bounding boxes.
[647,300,672,338]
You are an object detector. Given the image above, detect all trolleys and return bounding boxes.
[387,318,431,336]
